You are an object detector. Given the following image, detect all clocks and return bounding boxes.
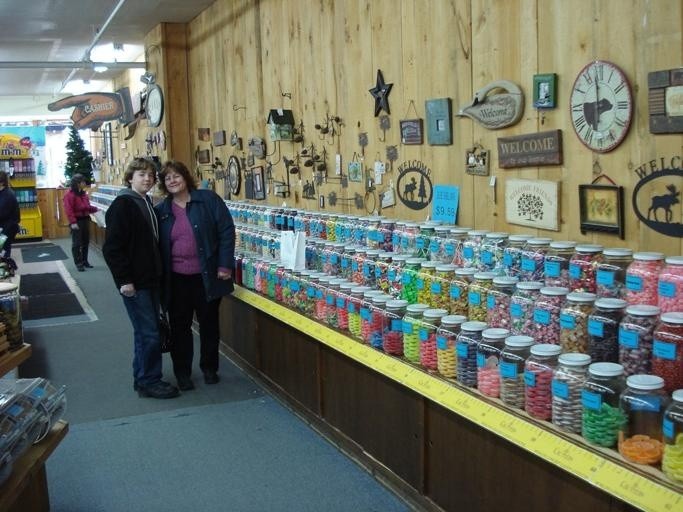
[569,59,634,152]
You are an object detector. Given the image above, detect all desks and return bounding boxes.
[0,342,33,378]
[1,419,70,512]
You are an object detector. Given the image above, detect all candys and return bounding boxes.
[626,260,666,306]
[652,320,683,394]
[618,315,661,377]
[663,434,683,483]
[658,264,683,313]
[569,253,604,294]
[618,430,663,466]
[280,208,359,339]
[226,199,280,302]
[597,260,634,298]
[460,227,587,436]
[581,402,628,448]
[587,307,628,362]
[359,216,460,380]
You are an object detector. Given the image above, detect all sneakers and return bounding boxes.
[133,378,170,390]
[203,365,219,385]
[136,379,178,398]
[81,260,93,268]
[75,261,84,272]
[173,371,194,391]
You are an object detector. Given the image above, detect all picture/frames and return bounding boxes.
[227,155,240,195]
[144,85,164,127]
[646,68,682,134]
[578,184,625,237]
[104,123,113,166]
[398,117,424,146]
[533,74,558,109]
[251,165,265,200]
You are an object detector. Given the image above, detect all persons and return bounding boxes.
[149,159,236,391]
[100,156,179,401]
[62,172,101,272]
[0,169,22,276]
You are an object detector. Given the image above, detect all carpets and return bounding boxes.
[20,246,68,263]
[19,292,87,322]
[19,272,71,294]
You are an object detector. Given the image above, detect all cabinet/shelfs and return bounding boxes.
[88,215,105,249]
[36,188,72,238]
[219,281,683,511]
[0,134,42,242]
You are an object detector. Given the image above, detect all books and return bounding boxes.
[0,318,10,363]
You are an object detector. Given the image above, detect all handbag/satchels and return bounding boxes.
[159,317,170,353]
